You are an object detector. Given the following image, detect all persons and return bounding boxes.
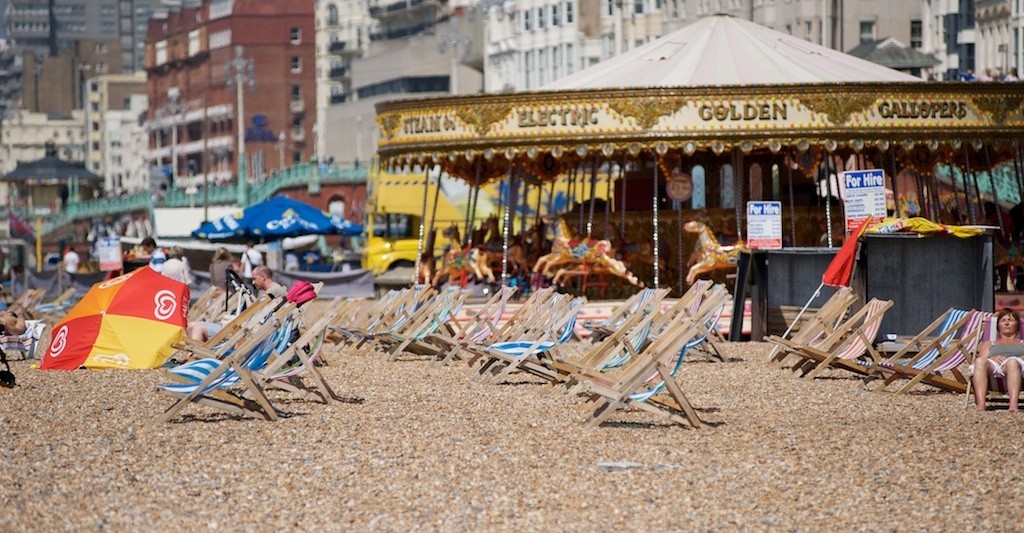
[959,67,1020,82]
[972,307,1024,411]
[0,216,362,362]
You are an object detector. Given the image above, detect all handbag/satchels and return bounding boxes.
[251,264,256,271]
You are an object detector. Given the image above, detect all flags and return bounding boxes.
[9,210,35,245]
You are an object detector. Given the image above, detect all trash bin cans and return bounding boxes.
[861,226,1000,350]
[748,246,840,342]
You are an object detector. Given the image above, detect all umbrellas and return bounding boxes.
[191,195,364,246]
[761,213,1001,357]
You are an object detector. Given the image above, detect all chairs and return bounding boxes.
[0,286,78,382]
[768,286,1024,407]
[153,275,738,428]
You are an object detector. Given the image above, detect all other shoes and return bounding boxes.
[171,359,188,367]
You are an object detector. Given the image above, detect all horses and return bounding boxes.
[425,215,749,290]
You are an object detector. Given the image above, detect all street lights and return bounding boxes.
[222,45,256,211]
[165,86,181,187]
